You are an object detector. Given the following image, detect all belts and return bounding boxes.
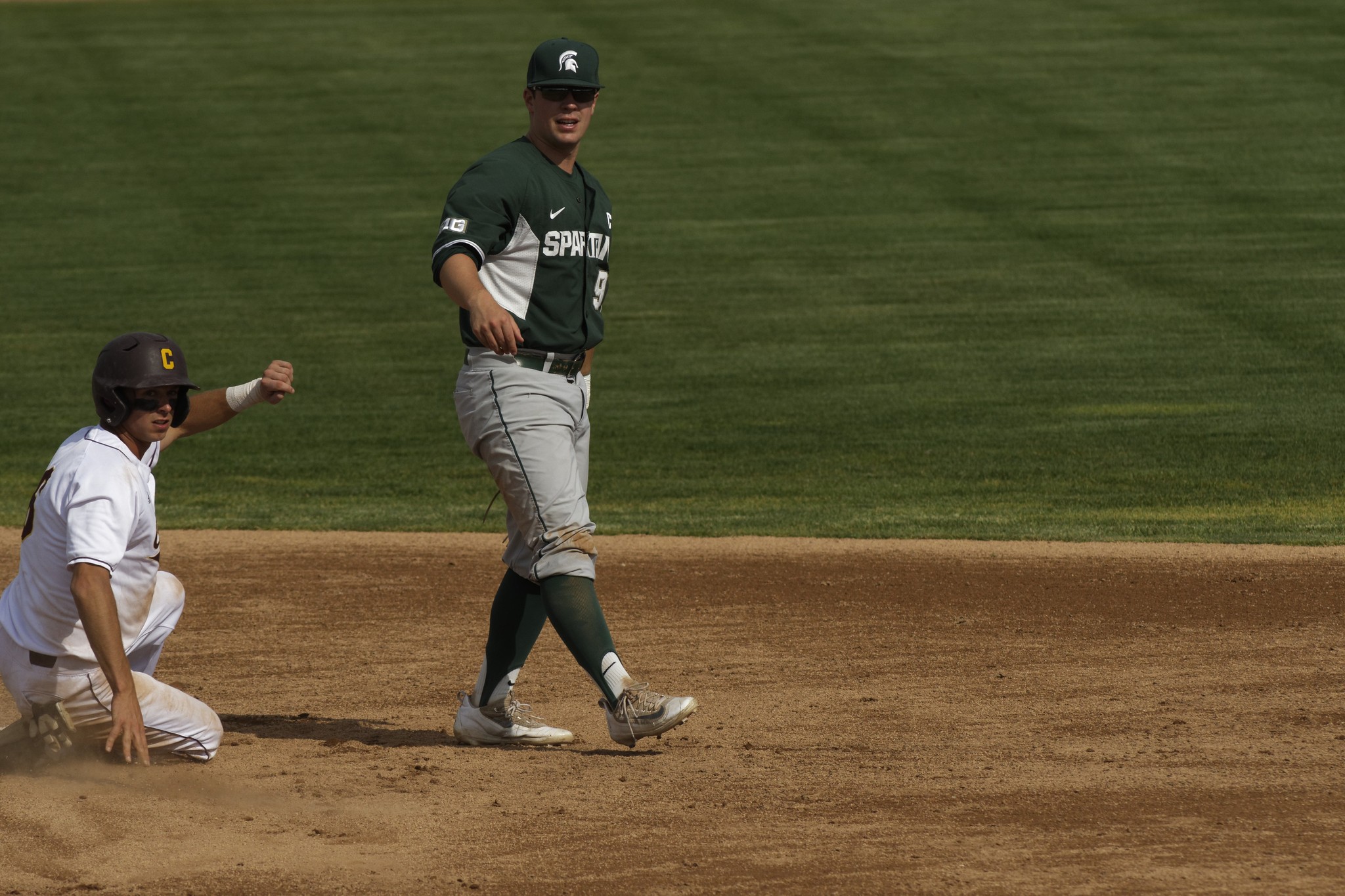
[463,348,586,377]
[29,651,57,668]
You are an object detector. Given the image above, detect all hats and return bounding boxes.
[527,36,606,89]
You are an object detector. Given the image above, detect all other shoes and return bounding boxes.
[1,718,38,757]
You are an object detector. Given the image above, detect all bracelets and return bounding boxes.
[226,377,268,412]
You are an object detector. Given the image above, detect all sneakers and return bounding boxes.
[598,680,698,749]
[453,690,573,747]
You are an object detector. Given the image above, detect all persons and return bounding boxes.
[0,330,295,767]
[431,38,698,749]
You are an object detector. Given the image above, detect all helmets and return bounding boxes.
[91,332,200,428]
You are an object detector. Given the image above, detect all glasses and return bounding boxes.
[529,85,598,103]
